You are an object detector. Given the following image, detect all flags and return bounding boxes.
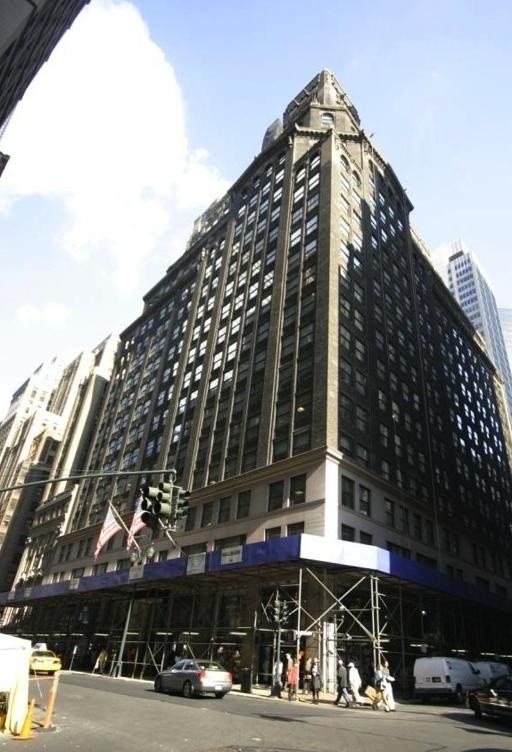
[93,498,125,564]
[125,487,156,553]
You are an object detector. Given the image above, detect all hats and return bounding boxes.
[309,658,320,664]
[337,660,355,667]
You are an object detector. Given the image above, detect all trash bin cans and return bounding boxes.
[240,667,251,694]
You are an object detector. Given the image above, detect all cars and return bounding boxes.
[464,674,512,723]
[29,642,63,677]
[153,659,234,700]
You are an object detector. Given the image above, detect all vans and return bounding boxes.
[474,661,509,687]
[412,656,492,706]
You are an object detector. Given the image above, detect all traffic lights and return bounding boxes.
[151,482,173,518]
[170,484,191,521]
[281,602,289,626]
[141,486,160,528]
[270,600,280,626]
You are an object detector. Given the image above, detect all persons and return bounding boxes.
[174,650,183,663]
[270,650,397,713]
[99,645,108,674]
